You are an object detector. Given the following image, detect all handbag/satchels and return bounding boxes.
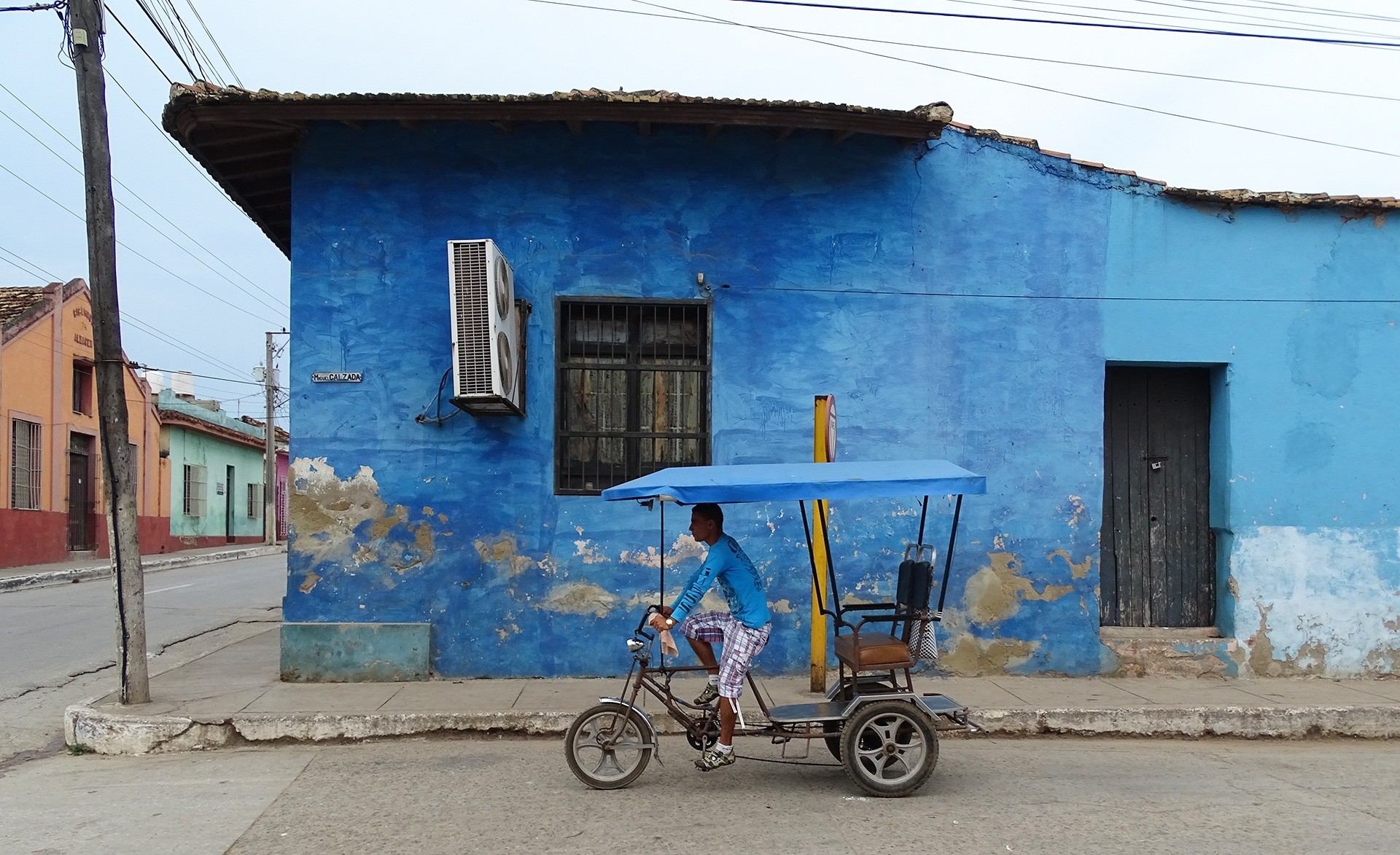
[908,606,938,658]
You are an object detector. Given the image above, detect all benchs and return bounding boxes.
[833,543,936,713]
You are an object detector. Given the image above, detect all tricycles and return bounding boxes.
[561,456,988,798]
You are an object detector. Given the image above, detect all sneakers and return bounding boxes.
[694,746,735,769]
[694,682,720,705]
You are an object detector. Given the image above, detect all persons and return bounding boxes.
[648,502,772,770]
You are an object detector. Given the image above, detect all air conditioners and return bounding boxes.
[446,238,519,410]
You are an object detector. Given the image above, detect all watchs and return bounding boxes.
[665,617,673,629]
